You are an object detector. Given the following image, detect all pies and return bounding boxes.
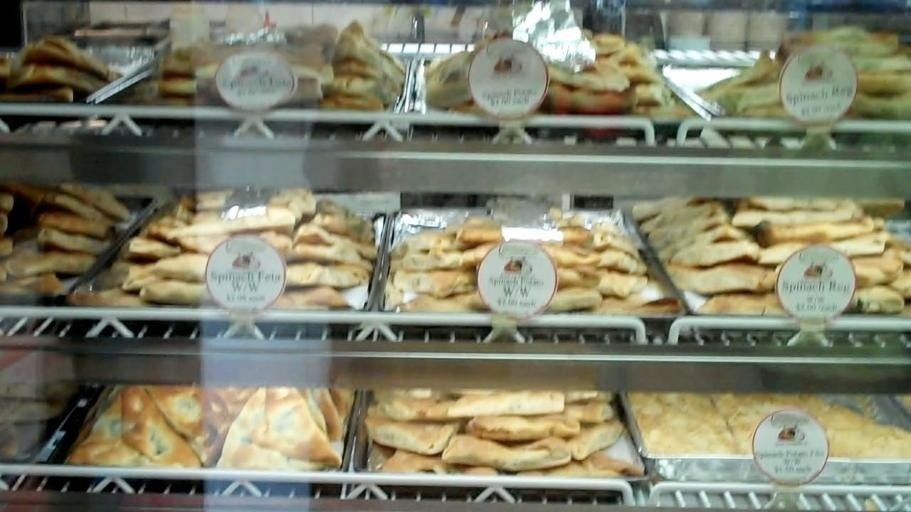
[0,21,911,486]
